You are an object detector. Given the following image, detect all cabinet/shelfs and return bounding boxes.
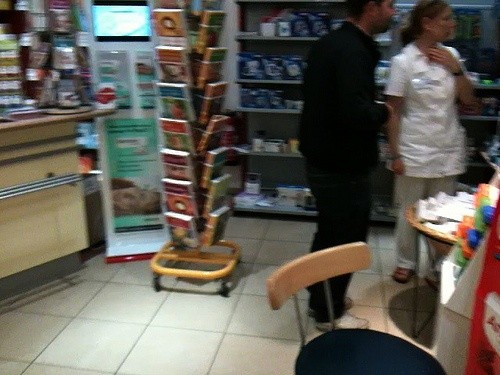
[233,0,500,224]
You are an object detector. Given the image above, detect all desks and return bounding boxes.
[405,202,463,338]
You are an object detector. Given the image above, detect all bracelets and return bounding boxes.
[453,68,464,76]
[389,155,401,160]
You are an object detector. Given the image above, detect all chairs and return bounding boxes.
[269,240,435,353]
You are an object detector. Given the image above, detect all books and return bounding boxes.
[154,45,193,87]
[204,207,232,246]
[197,115,231,154]
[198,81,228,124]
[204,173,232,218]
[202,146,230,188]
[197,47,228,88]
[155,82,196,121]
[196,11,227,55]
[161,178,199,220]
[158,118,195,157]
[152,8,192,54]
[164,211,198,249]
[159,147,197,187]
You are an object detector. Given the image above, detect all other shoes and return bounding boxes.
[393,267,415,282]
[315,312,370,331]
[308,297,354,317]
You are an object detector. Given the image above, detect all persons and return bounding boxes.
[297,0,394,329]
[383,0,473,292]
